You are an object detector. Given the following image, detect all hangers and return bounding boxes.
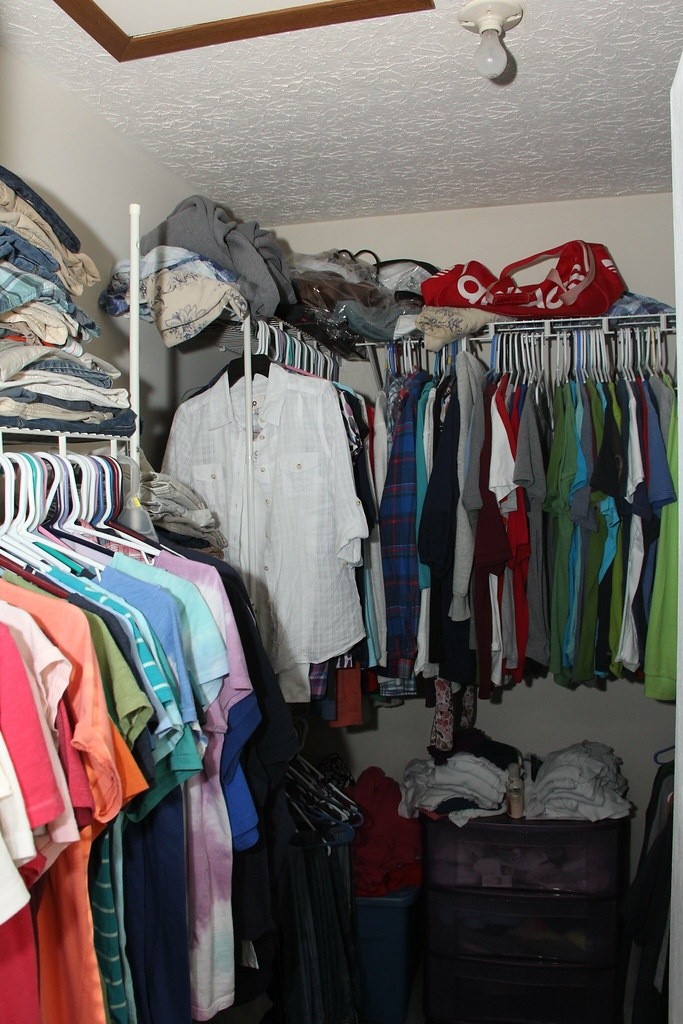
[279,746,367,856]
[1,447,239,631]
[180,314,677,422]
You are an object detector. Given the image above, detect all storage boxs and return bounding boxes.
[420,799,634,1024]
[352,882,418,1024]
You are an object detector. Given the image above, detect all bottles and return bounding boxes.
[504,762,523,819]
